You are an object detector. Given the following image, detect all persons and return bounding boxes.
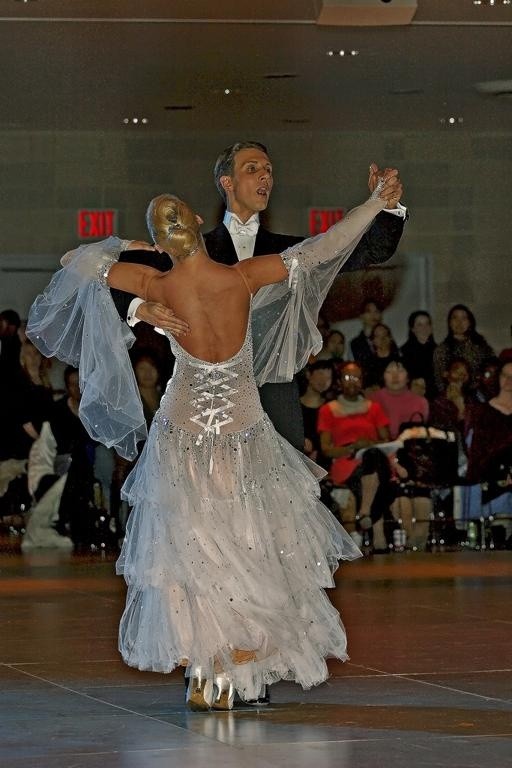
[0,292,511,553]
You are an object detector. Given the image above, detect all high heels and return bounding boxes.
[186,671,213,712]
[212,673,237,710]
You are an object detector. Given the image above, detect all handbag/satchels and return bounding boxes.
[400,412,432,431]
[405,424,459,485]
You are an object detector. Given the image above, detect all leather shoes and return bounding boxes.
[237,691,270,707]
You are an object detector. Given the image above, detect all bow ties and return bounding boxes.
[228,216,260,236]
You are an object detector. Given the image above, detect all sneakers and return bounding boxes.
[343,513,512,551]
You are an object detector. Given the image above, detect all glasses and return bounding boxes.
[342,374,361,383]
